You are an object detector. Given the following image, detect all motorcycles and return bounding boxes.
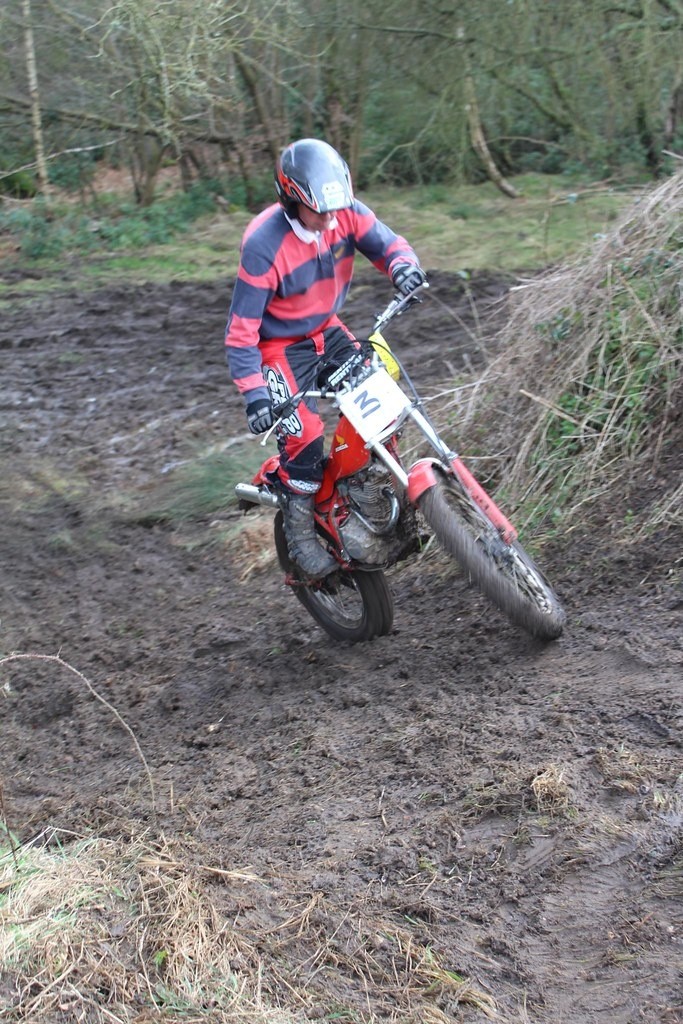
[227,282,570,646]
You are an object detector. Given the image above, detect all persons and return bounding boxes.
[224,138,431,583]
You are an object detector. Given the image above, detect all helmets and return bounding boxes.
[274,138,355,220]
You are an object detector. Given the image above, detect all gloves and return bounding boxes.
[391,262,426,300]
[246,399,276,435]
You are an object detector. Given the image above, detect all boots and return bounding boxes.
[283,493,340,579]
